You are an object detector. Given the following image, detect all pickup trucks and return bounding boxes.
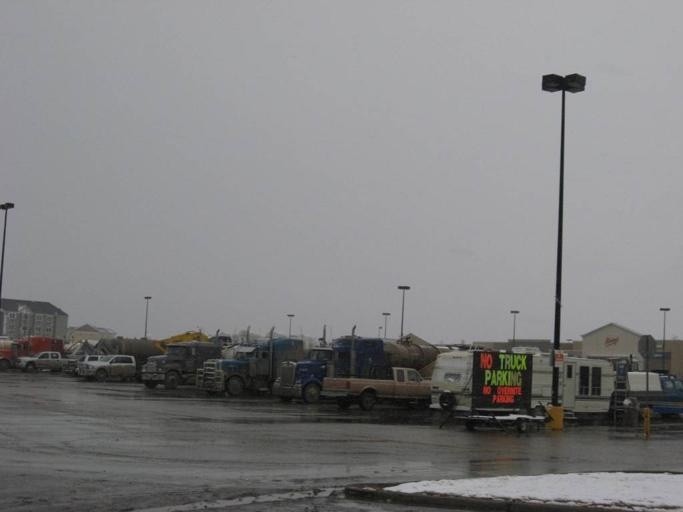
[16,350,78,373]
[319,366,432,405]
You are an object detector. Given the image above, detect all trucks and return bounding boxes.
[199,337,303,398]
[269,334,439,404]
[433,348,683,425]
[140,341,223,390]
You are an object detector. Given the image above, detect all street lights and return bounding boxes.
[142,295,151,338]
[508,310,520,340]
[0,202,14,310]
[381,311,391,336]
[285,314,295,338]
[396,285,410,336]
[537,68,588,410]
[660,307,671,371]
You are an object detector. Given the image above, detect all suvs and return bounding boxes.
[80,354,104,366]
[78,354,137,379]
